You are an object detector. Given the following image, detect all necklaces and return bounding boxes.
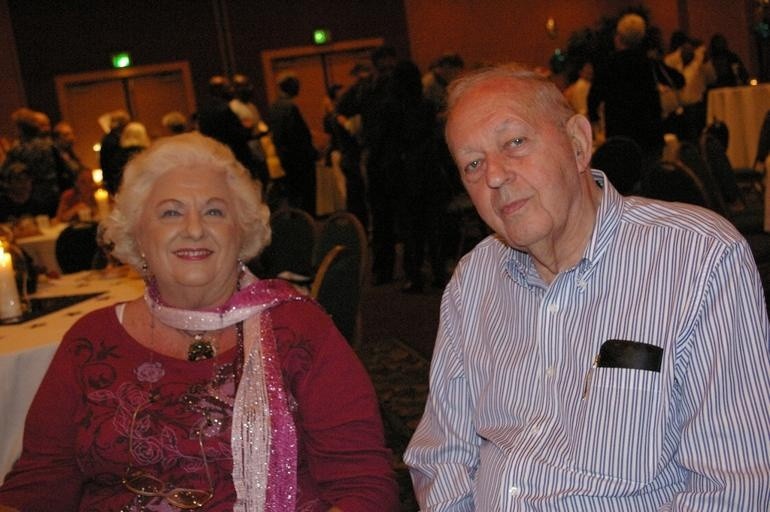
[183,329,216,362]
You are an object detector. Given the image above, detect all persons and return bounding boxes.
[404,61,770,512]
[0,131,404,511]
[2,15,769,297]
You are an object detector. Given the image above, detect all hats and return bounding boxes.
[119,122,152,149]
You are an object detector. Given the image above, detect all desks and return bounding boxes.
[0,218,147,482]
[700,81,770,168]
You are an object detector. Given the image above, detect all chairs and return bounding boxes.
[265,205,370,362]
